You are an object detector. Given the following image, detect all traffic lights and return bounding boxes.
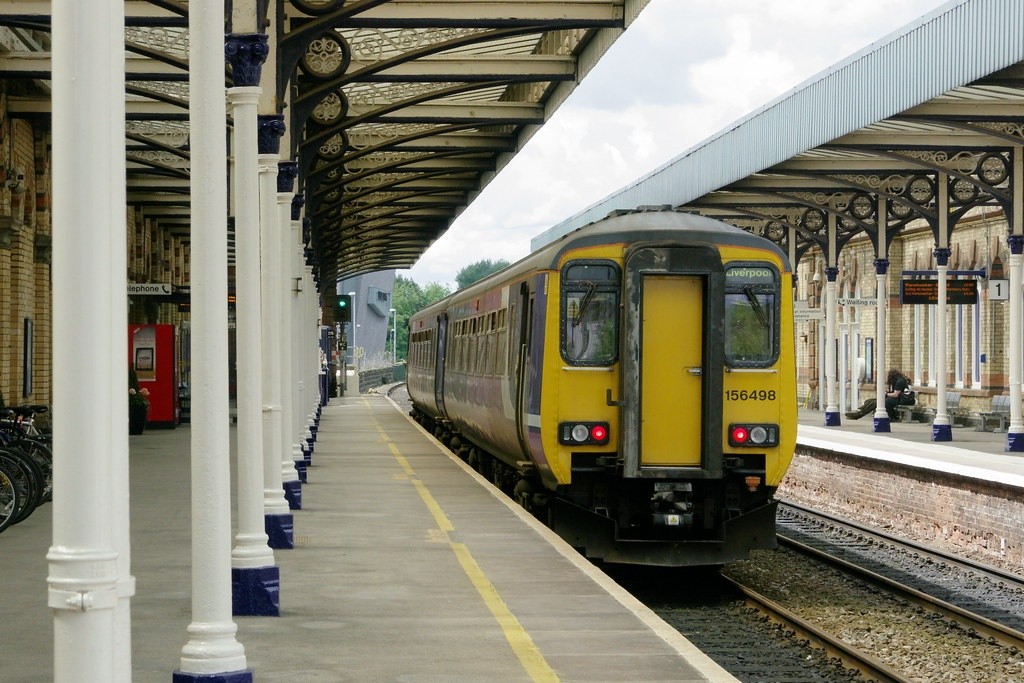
[332,293,353,323]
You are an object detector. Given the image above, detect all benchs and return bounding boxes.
[979,395,1010,433]
[894,391,920,423]
[922,392,962,427]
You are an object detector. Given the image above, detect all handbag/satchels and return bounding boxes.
[898,391,915,405]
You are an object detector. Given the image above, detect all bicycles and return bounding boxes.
[0,403,53,534]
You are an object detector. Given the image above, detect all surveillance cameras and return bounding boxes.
[12,167,24,182]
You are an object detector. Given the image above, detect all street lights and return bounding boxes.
[389,328,395,353]
[347,291,356,366]
[388,308,397,364]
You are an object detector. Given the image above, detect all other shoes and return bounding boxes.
[891,415,899,422]
[845,413,859,420]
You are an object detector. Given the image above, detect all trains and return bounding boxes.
[403,204,801,569]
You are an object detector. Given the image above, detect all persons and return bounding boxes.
[845,369,914,422]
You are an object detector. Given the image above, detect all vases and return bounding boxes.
[129,410,148,435]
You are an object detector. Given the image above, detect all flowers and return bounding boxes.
[129,388,151,411]
